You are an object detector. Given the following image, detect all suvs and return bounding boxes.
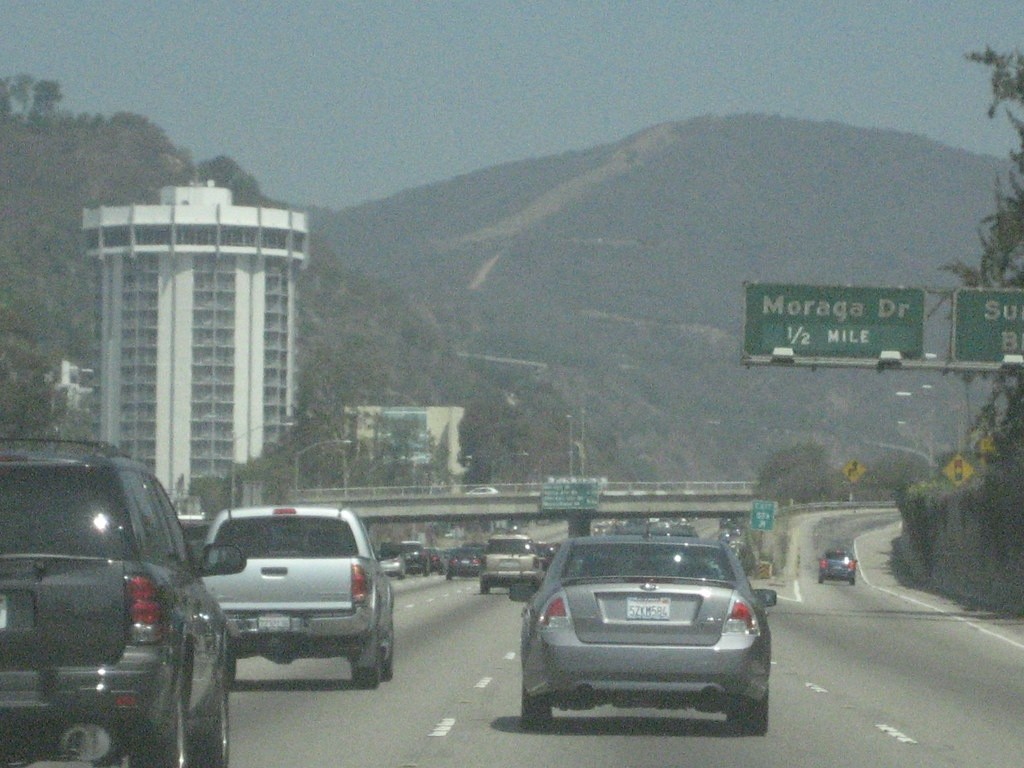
[818,549,857,584]
[481,535,541,593]
[0,435,247,768]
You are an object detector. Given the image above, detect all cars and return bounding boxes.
[593,520,699,539]
[465,487,497,494]
[375,541,448,580]
[510,537,776,737]
[720,518,748,554]
[175,516,213,561]
[445,543,485,577]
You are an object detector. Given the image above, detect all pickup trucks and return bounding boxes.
[199,505,395,687]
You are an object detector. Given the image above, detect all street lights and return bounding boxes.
[295,439,352,501]
